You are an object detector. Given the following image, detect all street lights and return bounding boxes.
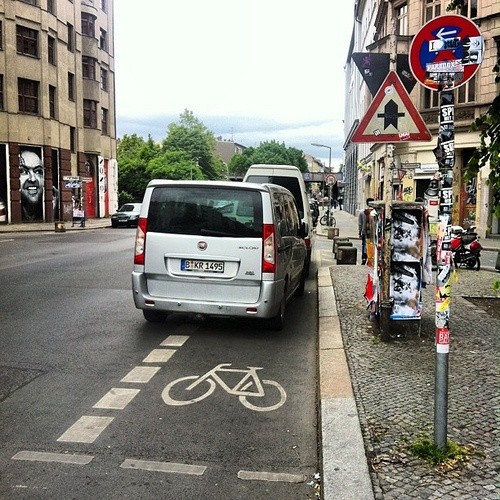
[311,143,331,226]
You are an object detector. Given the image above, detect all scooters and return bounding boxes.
[429,226,481,270]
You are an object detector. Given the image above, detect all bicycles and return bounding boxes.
[320,207,336,226]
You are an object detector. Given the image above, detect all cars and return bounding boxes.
[110,203,142,228]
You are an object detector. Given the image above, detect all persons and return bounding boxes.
[305,190,345,223]
[19,150,44,223]
[358,198,377,266]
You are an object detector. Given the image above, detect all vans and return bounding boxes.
[130,164,314,330]
